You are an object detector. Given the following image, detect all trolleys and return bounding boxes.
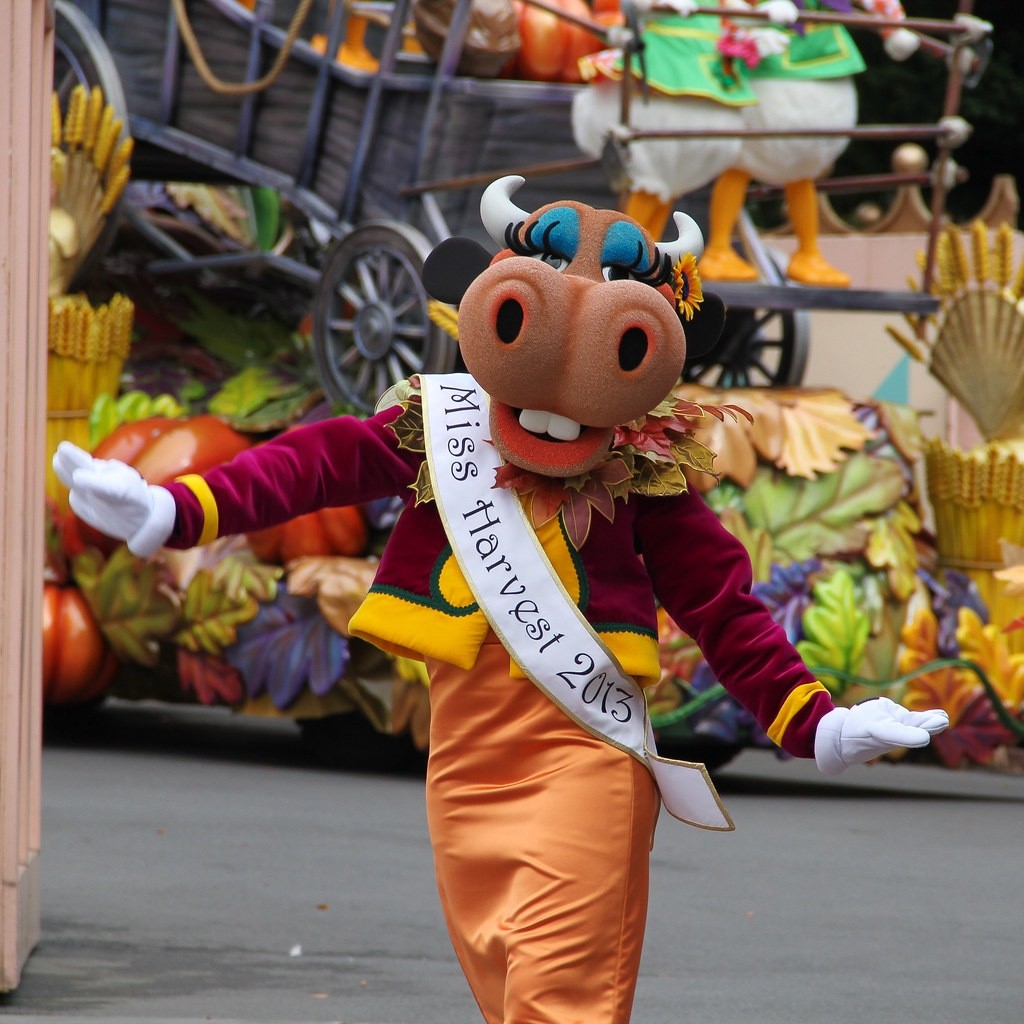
[52,0,995,424]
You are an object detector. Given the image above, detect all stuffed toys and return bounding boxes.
[696,1,875,289]
[570,0,789,280]
[47,174,950,1024]
